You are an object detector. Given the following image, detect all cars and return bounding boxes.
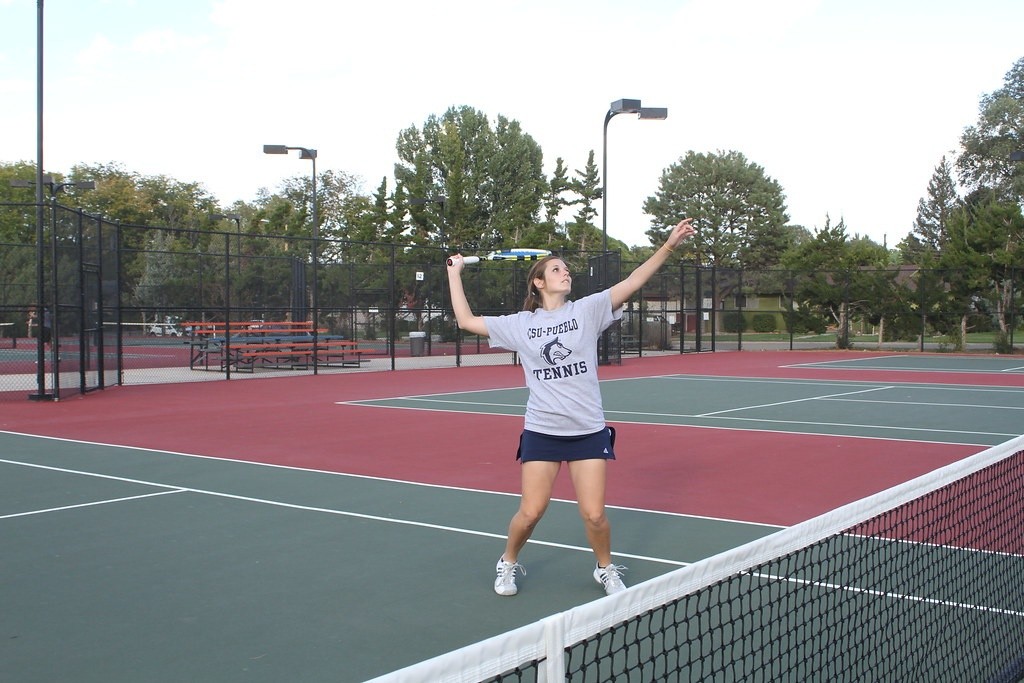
[148,316,196,338]
[242,320,264,329]
[210,213,244,320]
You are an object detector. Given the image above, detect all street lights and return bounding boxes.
[263,144,318,375]
[602,99,669,365]
[8,179,95,402]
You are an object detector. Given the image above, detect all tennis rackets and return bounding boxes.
[447,248,552,264]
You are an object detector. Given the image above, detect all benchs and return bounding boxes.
[181,321,376,373]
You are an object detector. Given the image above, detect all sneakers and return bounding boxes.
[494,556,529,597]
[591,561,627,596]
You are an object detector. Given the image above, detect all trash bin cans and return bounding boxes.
[409,331,426,357]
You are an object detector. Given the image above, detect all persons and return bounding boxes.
[30,308,62,364]
[444,215,697,600]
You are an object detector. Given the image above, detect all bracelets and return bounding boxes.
[662,243,674,254]
